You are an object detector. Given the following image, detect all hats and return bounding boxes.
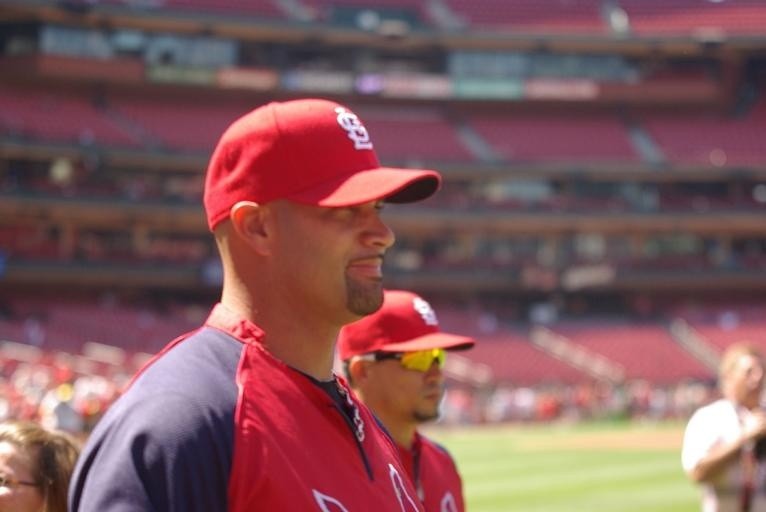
[336,289,475,361]
[204,98,443,232]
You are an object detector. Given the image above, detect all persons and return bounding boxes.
[1,2,765,433]
[334,288,479,512]
[66,97,445,510]
[1,418,84,511]
[677,341,766,511]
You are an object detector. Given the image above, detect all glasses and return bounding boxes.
[1,472,42,489]
[350,349,447,371]
